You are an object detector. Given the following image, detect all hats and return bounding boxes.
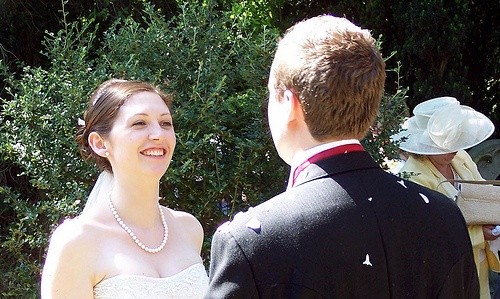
[389,96,494,155]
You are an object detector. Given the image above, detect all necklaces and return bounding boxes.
[107,194,168,253]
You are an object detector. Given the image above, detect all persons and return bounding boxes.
[210,16,481,299]
[390,96,500,299]
[40,80,210,299]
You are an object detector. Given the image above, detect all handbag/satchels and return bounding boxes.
[435,180,500,224]
